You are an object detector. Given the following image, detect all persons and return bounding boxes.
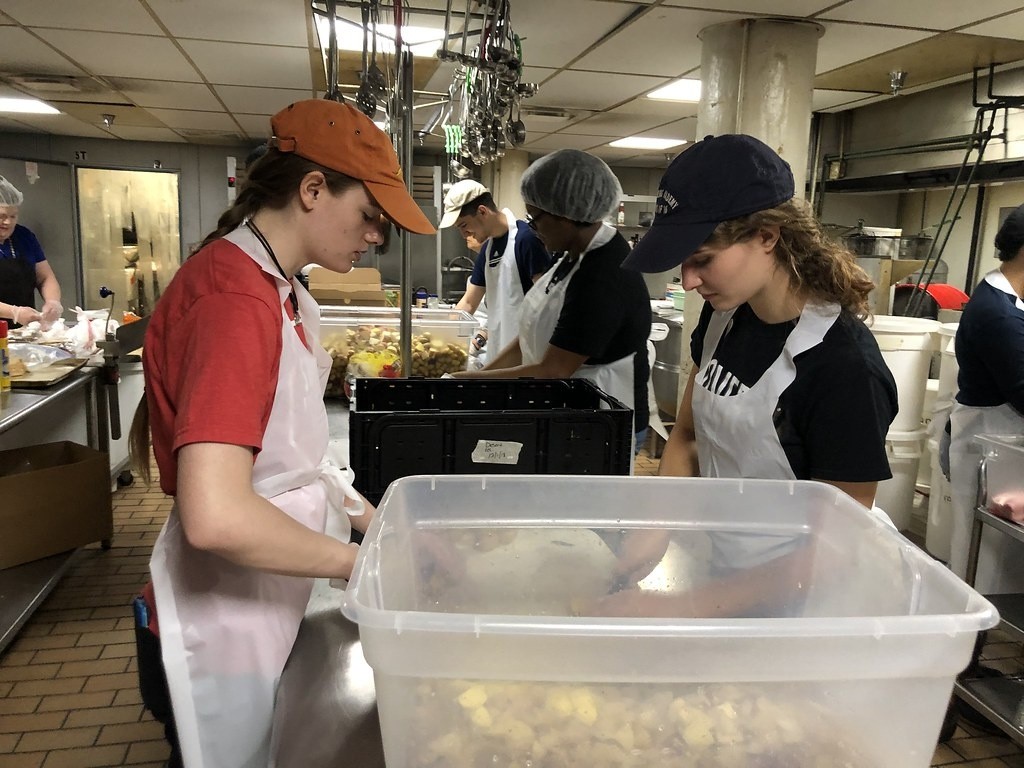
[925,205,1024,734]
[571,135,898,620]
[439,179,553,364]
[0,176,61,329]
[127,99,444,768]
[442,149,651,456]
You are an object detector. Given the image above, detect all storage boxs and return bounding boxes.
[318,305,480,398]
[308,267,387,306]
[341,474,1001,768]
[350,377,633,546]
[973,435,1024,527]
[0,441,114,569]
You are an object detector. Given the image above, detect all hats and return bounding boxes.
[269,99,438,235]
[994,204,1024,251]
[439,179,490,229]
[621,133,794,274]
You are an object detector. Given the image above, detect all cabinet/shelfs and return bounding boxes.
[937,457,1024,748]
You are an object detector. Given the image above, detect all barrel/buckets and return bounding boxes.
[853,314,939,429]
[932,319,961,431]
[926,440,954,559]
[911,380,940,493]
[868,430,926,533]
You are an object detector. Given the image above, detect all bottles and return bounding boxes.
[618,202,625,223]
[427,295,439,313]
[0,321,10,391]
[416,292,427,318]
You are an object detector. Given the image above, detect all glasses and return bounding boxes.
[526,211,546,231]
